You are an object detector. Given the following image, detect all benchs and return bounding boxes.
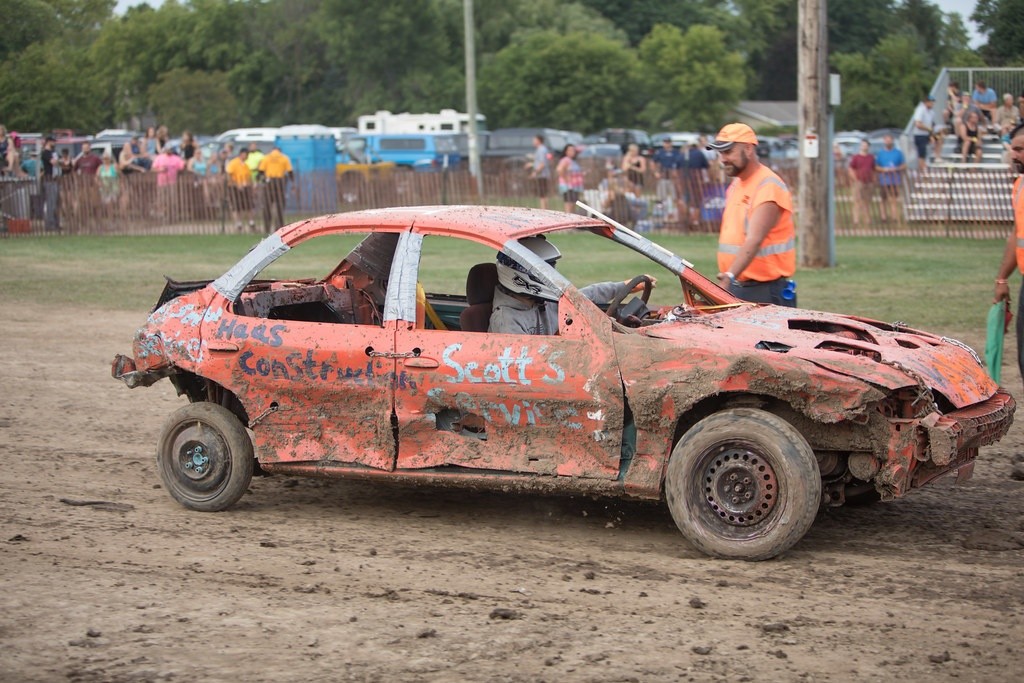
[585,189,637,217]
[906,117,1024,221]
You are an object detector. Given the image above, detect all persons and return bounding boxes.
[848,139,874,225]
[991,123,1024,384]
[622,142,646,191]
[651,138,710,206]
[706,123,799,308]
[875,135,907,224]
[524,134,551,210]
[492,238,658,334]
[0,125,297,235]
[555,145,584,211]
[911,71,1024,169]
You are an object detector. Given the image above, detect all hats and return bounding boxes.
[925,96,935,102]
[705,122,758,152]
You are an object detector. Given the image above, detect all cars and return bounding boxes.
[4,107,901,174]
[108,199,1018,562]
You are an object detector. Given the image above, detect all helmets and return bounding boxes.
[496,240,563,305]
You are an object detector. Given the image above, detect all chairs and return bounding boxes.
[460,263,499,333]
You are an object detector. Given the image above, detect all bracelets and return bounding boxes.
[727,272,735,283]
[994,279,1009,284]
